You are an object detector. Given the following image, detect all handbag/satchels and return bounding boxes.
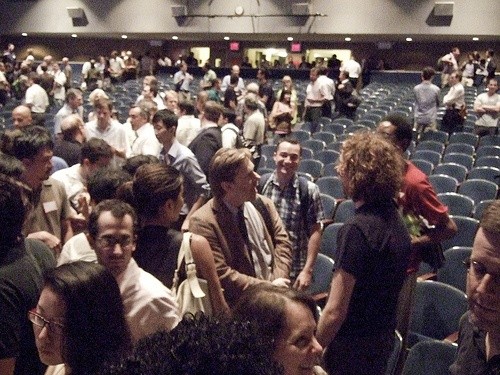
[171,232,214,317]
[267,101,280,129]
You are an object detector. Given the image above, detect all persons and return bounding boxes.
[0,27,387,375]
[447,199,500,374]
[314,130,412,374]
[373,116,458,276]
[413,42,500,138]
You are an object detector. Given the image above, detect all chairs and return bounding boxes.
[0,73,500,375]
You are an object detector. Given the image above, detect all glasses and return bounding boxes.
[28,309,65,335]
[462,257,500,288]
[95,237,133,247]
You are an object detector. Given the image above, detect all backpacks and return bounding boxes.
[341,83,362,113]
[221,127,261,172]
